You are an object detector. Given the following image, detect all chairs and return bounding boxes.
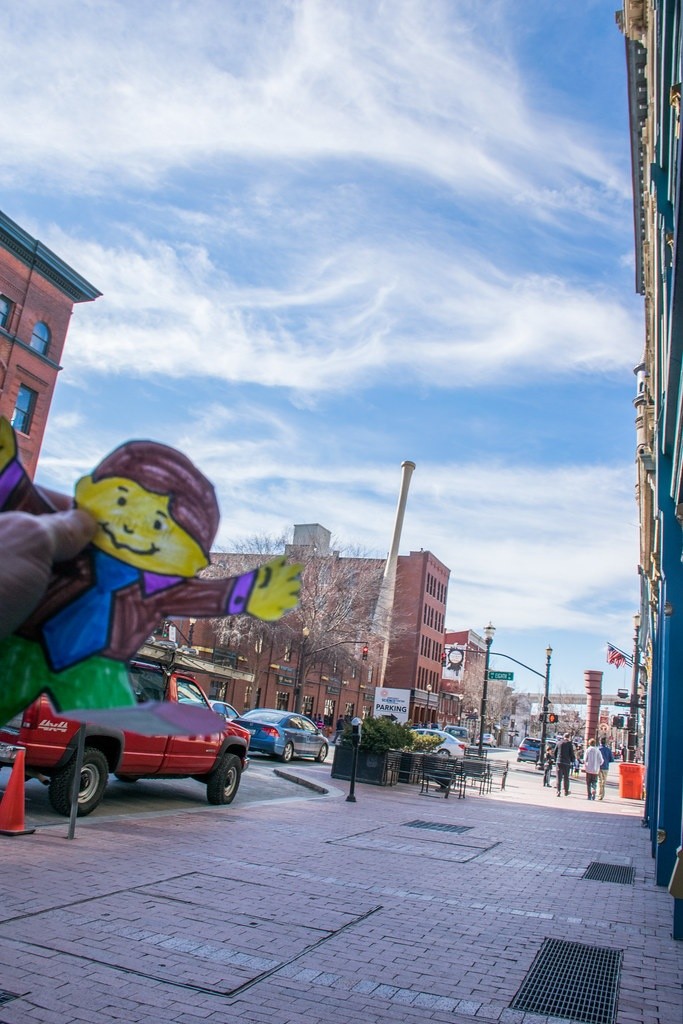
[384,747,509,799]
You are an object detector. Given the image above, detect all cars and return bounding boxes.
[475,733,498,748]
[174,696,242,723]
[229,708,330,762]
[408,728,469,759]
[516,733,586,762]
[442,725,472,746]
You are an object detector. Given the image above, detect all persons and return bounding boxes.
[543,746,554,787]
[584,739,604,800]
[331,715,345,743]
[597,737,614,800]
[312,713,325,729]
[570,749,584,777]
[622,746,626,762]
[418,721,438,729]
[553,733,573,797]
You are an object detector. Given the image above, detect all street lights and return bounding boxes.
[422,685,435,726]
[295,625,311,716]
[186,617,198,646]
[625,612,641,764]
[477,621,498,756]
[536,644,553,770]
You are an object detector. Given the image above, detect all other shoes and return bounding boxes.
[564,791,571,796]
[556,791,561,797]
[587,791,605,800]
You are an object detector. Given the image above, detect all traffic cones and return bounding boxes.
[0,750,39,836]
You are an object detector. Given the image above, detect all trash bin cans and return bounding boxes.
[619,763,645,801]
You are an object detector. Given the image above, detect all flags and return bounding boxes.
[607,644,626,668]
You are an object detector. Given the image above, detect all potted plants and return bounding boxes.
[331,717,447,786]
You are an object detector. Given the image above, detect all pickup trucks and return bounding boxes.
[0,660,251,821]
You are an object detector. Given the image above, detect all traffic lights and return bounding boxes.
[551,715,560,722]
[441,652,447,667]
[162,621,169,638]
[362,646,369,661]
[154,621,162,634]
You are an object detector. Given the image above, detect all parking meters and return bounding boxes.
[345,717,363,803]
